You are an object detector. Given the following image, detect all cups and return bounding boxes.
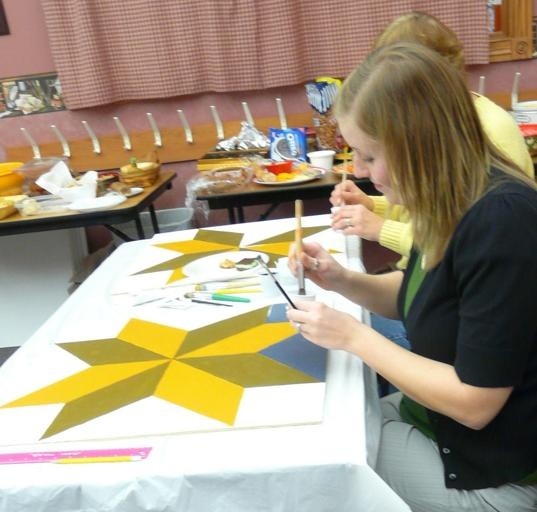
[307,150,336,172]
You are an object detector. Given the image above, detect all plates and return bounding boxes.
[181,249,268,283]
[253,167,326,187]
[103,187,144,198]
[68,196,128,211]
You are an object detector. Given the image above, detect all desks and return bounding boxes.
[189,146,537,224]
[0,215,368,512]
[1,171,179,240]
[0,110,536,240]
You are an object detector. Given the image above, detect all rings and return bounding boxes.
[344,218,351,225]
[312,258,320,269]
[296,322,302,331]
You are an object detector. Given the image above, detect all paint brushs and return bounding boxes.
[184,281,262,306]
[342,146,347,207]
[294,199,306,295]
[253,255,295,308]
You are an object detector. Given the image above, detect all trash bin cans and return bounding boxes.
[111,208,197,245]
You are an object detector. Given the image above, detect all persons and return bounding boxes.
[286,40,537,512]
[325,8,537,397]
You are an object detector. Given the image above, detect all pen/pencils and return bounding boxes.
[49,456,143,463]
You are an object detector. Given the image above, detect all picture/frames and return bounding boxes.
[1,71,63,119]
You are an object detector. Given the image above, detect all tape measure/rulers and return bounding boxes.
[0,447,153,464]
